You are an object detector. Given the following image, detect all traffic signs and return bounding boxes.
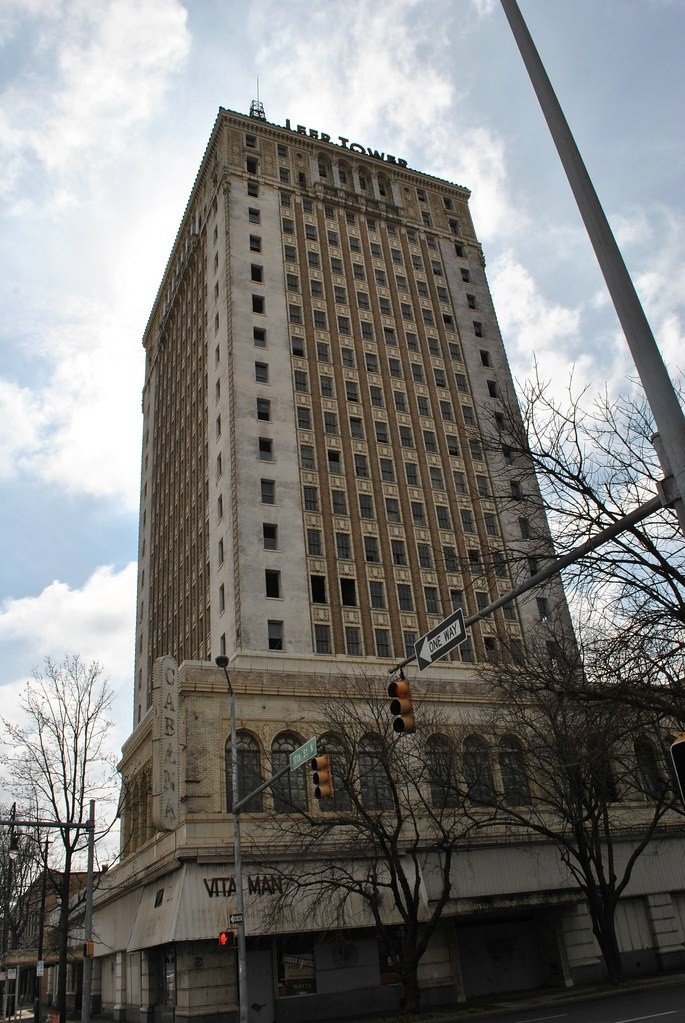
[229,914,243,924]
[413,607,467,672]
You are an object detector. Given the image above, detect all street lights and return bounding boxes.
[6,831,55,1023]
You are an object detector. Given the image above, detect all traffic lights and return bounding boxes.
[219,931,234,946]
[387,679,418,732]
[311,754,334,801]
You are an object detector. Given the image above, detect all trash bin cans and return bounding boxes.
[48,1010,60,1023]
[2,993,15,1016]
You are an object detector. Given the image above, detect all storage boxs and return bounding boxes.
[284,954,315,979]
